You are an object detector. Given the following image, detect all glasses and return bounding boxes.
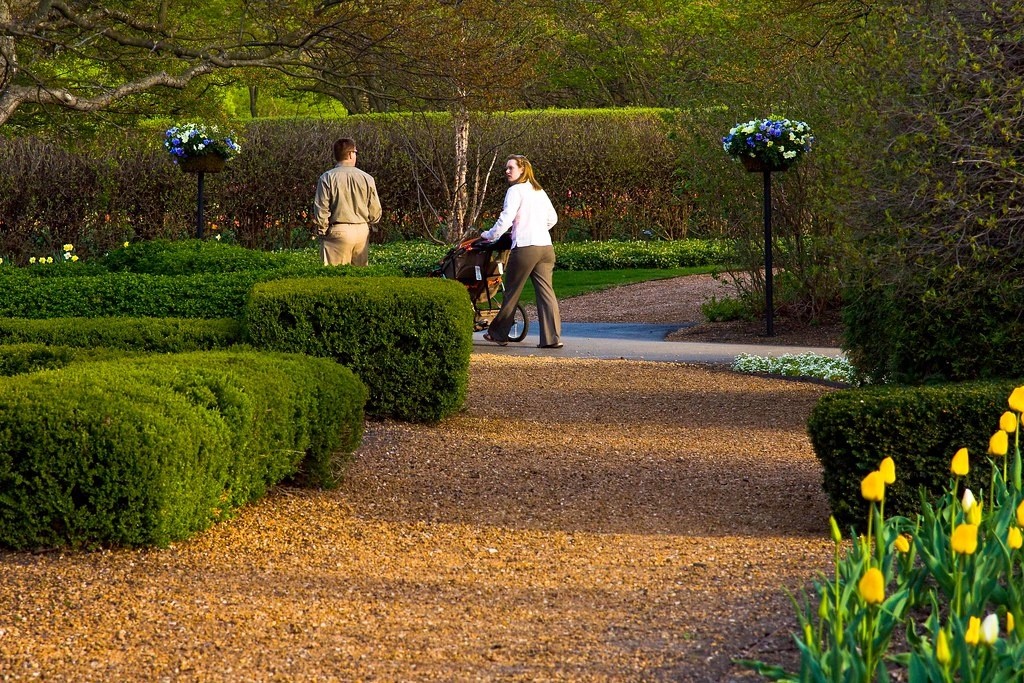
[349,149,358,155]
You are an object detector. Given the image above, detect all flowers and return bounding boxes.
[722,114,814,165]
[164,122,242,165]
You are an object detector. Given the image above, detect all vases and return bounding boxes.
[738,153,792,172]
[176,152,225,173]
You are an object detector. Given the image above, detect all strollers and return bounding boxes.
[425,230,530,343]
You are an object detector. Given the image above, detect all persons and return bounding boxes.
[481,154,564,349]
[312,139,382,266]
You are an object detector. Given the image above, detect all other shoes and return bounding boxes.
[537,340,563,348]
[483,333,509,346]
